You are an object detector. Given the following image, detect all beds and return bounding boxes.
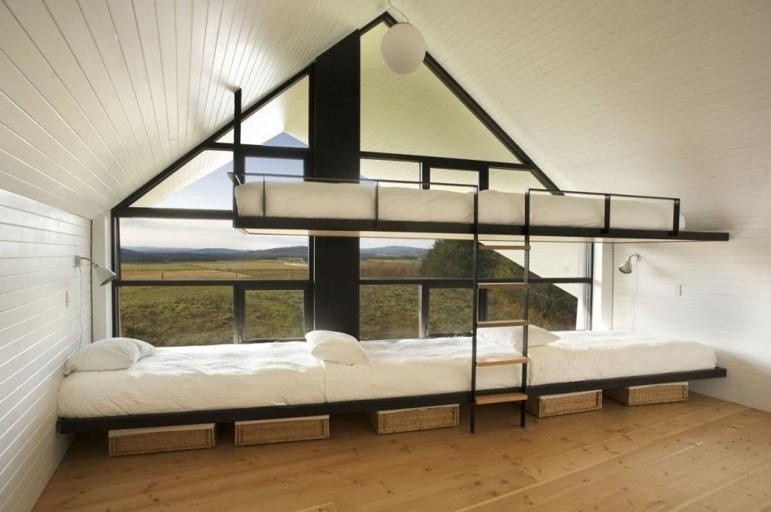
[231,174,731,241]
[56,327,726,431]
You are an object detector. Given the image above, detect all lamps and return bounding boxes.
[619,252,640,274]
[380,0,425,73]
[74,253,120,288]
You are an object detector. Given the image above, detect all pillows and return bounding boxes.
[469,319,560,350]
[64,335,155,373]
[304,329,363,365]
